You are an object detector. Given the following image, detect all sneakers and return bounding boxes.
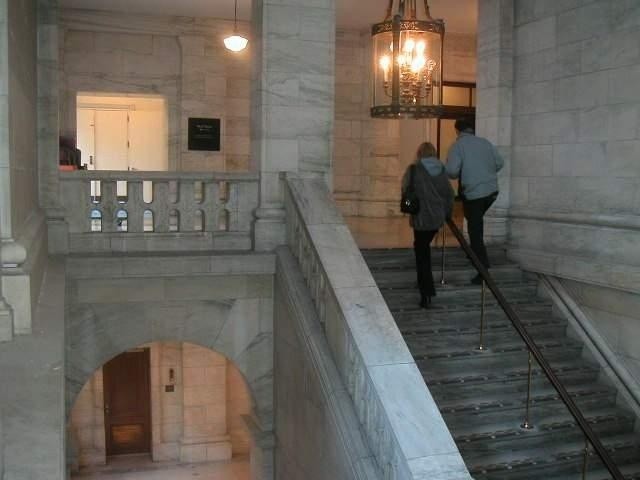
[419,288,436,308]
[471,260,491,285]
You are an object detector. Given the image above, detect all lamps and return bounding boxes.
[224,0,248,52]
[370,1,445,117]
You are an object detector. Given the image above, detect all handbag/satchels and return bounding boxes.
[400,190,420,214]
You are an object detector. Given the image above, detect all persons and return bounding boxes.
[443,116,504,284]
[400,142,453,307]
